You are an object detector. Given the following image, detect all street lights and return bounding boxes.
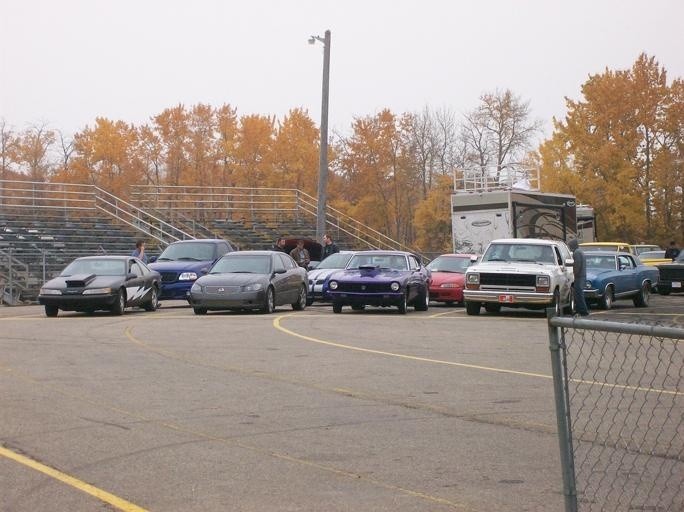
[305,28,335,258]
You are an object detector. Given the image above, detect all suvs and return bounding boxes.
[461,239,579,317]
[146,239,234,300]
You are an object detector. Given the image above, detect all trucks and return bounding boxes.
[450,192,596,254]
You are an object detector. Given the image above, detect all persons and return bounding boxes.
[289,239,311,272]
[271,237,289,254]
[319,232,339,262]
[664,241,680,262]
[566,237,590,318]
[131,239,148,266]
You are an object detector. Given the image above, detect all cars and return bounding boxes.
[304,252,367,306]
[573,241,684,310]
[322,250,431,313]
[35,254,162,317]
[418,252,485,307]
[185,250,312,313]
